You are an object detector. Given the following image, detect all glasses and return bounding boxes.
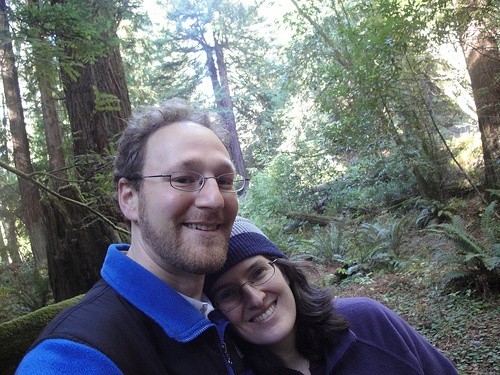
[143,170,252,196]
[213,258,280,314]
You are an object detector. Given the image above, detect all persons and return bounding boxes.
[204,215,461,375]
[13,97,256,375]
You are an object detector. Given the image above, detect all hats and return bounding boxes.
[203,215,289,290]
